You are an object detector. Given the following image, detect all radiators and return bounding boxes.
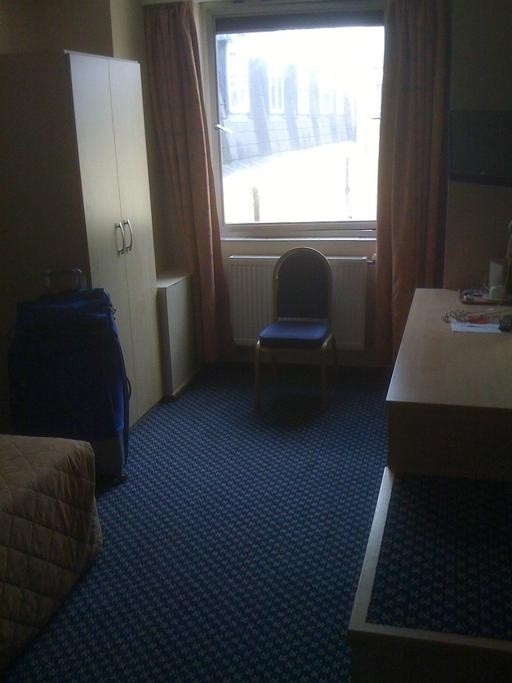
[226,254,368,355]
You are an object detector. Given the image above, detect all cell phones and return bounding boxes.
[498,314,512,330]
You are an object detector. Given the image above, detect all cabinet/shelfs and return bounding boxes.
[2,50,165,431]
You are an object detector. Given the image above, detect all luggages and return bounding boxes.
[8,268,132,483]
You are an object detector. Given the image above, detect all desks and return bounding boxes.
[385,285,510,486]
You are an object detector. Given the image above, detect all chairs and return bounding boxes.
[249,245,337,411]
